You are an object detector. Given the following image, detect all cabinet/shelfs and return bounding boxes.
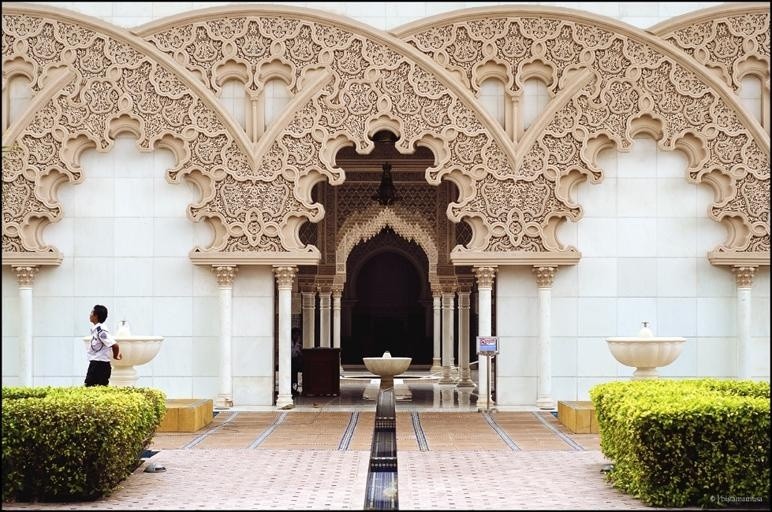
[300,347,340,398]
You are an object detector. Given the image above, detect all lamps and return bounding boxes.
[370,161,403,206]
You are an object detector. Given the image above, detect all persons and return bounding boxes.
[84,304,123,387]
